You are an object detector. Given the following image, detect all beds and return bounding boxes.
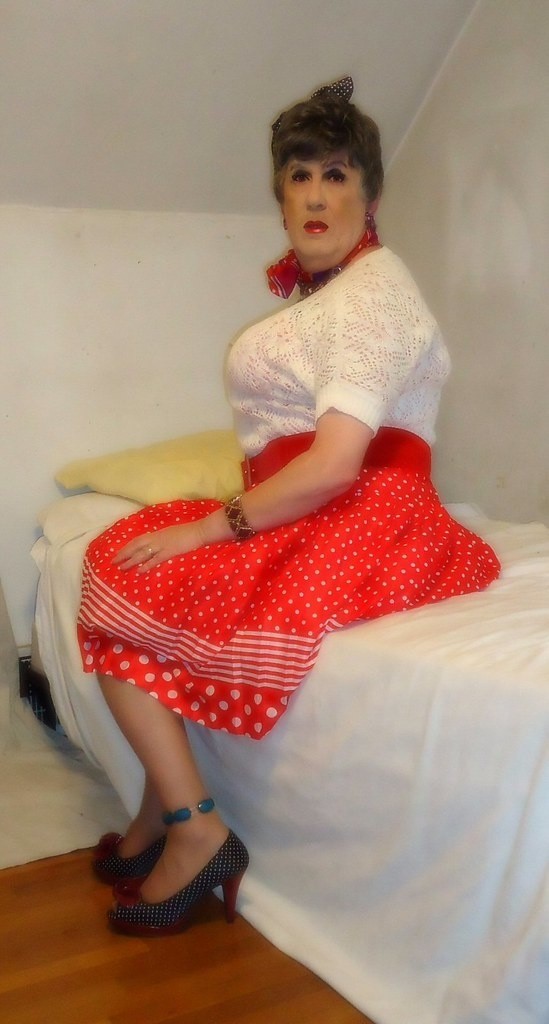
[28,491,548,1023]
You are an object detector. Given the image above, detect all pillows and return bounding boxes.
[54,428,244,502]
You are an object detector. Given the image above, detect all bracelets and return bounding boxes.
[225,496,255,540]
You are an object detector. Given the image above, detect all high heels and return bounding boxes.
[107,829,250,937]
[93,832,168,886]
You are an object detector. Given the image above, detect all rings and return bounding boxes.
[148,547,155,555]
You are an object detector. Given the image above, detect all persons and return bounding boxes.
[78,76,502,935]
